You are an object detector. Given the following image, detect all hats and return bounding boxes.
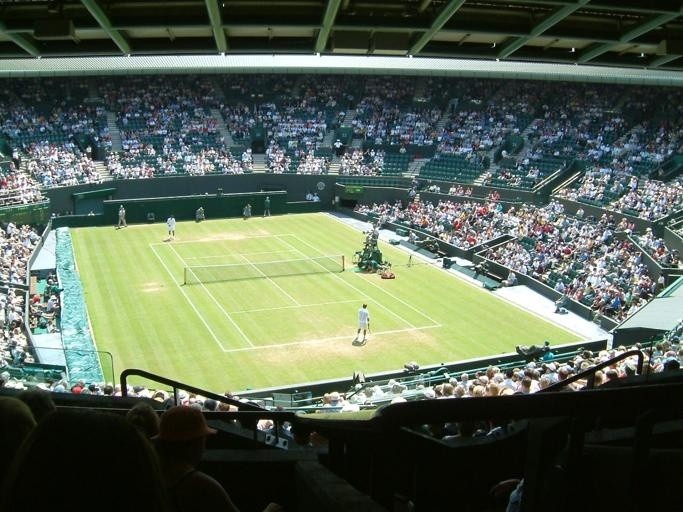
[330,392,339,400]
[519,371,525,378]
[480,376,488,382]
[546,362,573,375]
[665,351,677,362]
[147,404,217,442]
[527,362,536,368]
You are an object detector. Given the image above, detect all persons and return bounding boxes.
[2,327,681,512]
[0,216,65,334]
[242,201,252,221]
[166,214,176,240]
[350,215,681,325]
[114,204,128,231]
[195,206,205,223]
[262,195,271,217]
[2,71,682,215]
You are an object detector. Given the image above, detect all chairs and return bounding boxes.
[0,75,682,221]
[1,407,173,511]
